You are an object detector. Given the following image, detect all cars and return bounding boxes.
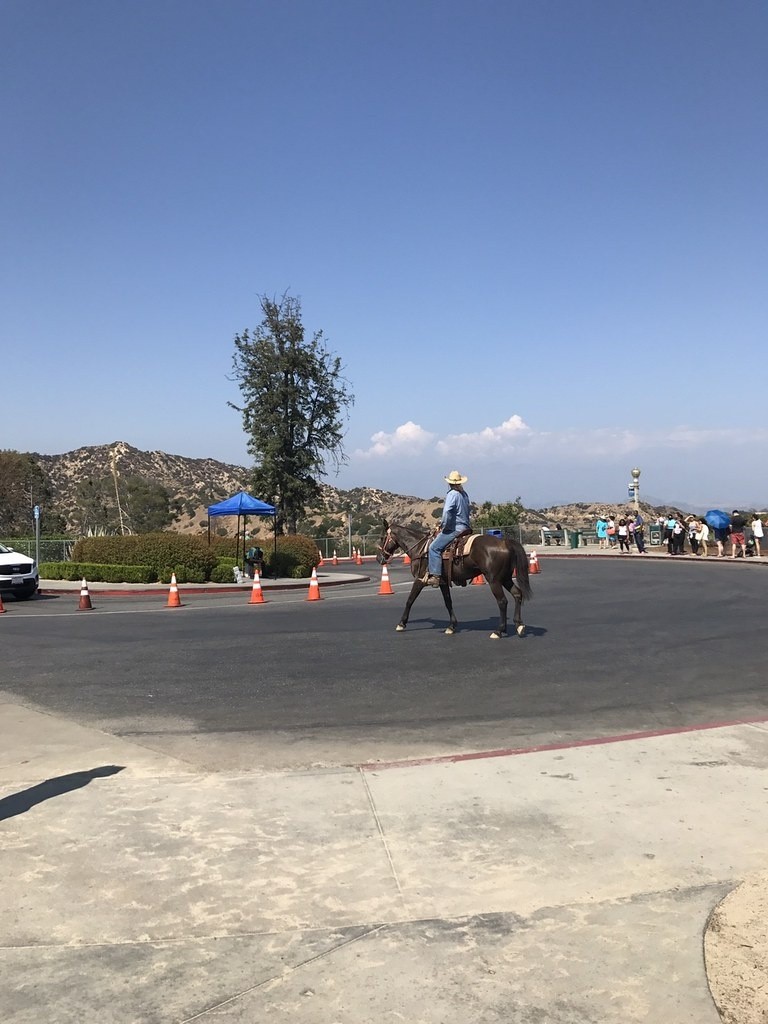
[0,542,40,600]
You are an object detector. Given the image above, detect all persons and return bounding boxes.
[419,471,470,589]
[712,526,731,557]
[541,523,563,546]
[684,513,700,556]
[671,515,687,556]
[655,512,681,555]
[596,509,646,555]
[728,510,747,559]
[751,514,764,557]
[695,517,710,557]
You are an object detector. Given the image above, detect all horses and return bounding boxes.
[376,518,534,640]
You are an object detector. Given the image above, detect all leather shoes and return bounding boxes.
[418,576,440,588]
[666,550,687,556]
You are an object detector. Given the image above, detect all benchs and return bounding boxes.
[577,528,598,546]
[539,528,571,546]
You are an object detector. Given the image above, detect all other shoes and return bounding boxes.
[690,553,696,555]
[717,554,723,557]
[743,556,746,558]
[729,556,735,558]
[627,550,631,554]
[618,549,622,553]
[701,552,707,557]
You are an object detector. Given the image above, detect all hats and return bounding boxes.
[601,515,606,519]
[443,471,467,484]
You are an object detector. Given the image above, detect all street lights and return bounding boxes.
[632,467,641,512]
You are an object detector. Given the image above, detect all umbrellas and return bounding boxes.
[706,510,730,529]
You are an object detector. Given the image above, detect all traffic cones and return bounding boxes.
[534,551,542,573]
[403,552,411,564]
[306,567,324,601]
[332,549,339,564]
[352,546,357,560]
[76,577,96,611]
[472,573,487,584]
[377,564,394,594]
[528,549,537,574]
[0,601,5,613]
[316,550,325,565]
[164,572,188,607]
[355,550,363,564]
[249,569,268,604]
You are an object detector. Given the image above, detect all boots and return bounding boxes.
[599,541,601,549]
[604,541,606,549]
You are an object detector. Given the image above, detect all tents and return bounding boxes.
[207,493,278,579]
